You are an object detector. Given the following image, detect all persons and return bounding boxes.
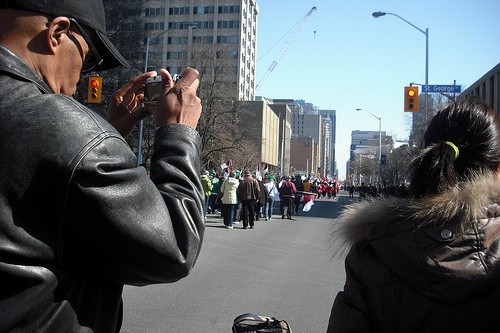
[220,171,240,229]
[238,171,260,229]
[342,182,408,201]
[200,169,339,223]
[256,175,269,221]
[279,176,297,220]
[326,94,500,333]
[0,0,206,333]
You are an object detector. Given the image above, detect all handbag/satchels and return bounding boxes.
[267,196,274,202]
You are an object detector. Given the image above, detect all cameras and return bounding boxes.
[143,73,182,102]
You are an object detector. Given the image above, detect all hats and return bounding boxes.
[287,176,292,179]
[0,0,132,75]
[202,169,209,175]
[269,175,273,180]
[245,171,251,175]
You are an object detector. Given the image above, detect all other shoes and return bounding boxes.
[204,208,224,220]
[242,226,254,229]
[232,211,301,224]
[225,224,233,228]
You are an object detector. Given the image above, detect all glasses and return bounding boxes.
[46,15,105,74]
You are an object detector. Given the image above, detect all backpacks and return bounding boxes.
[232,312,293,333]
